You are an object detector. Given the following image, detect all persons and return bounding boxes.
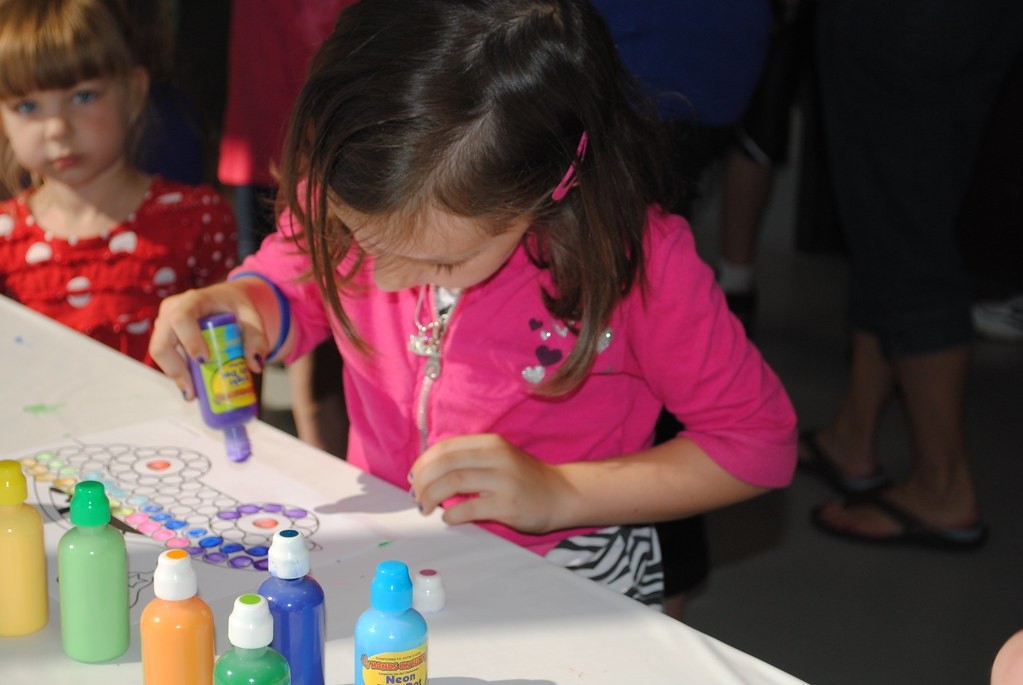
[0,0,239,372]
[142,0,1023,550]
[149,0,799,610]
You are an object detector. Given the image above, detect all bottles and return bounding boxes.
[186,310,258,463]
[57,479,131,662]
[354,561,427,685]
[0,459,49,637]
[211,593,290,685]
[257,528,326,685]
[140,549,214,685]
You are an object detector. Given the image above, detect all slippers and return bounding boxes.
[813,489,990,549]
[797,429,893,491]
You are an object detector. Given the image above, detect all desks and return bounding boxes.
[0,298,809,685]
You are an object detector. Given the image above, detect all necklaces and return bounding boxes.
[408,286,453,356]
